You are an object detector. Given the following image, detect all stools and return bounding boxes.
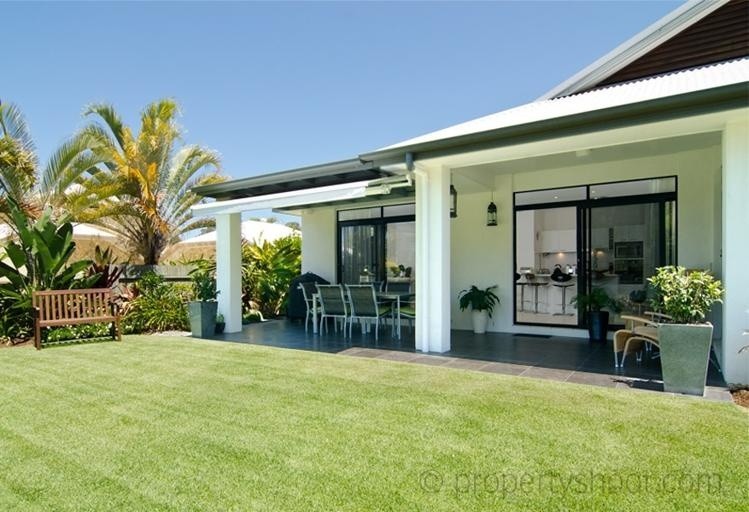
[516,268,609,318]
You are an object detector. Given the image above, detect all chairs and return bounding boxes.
[613,293,688,373]
[297,279,415,341]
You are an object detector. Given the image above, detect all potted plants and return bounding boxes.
[183,274,226,339]
[570,287,623,344]
[458,285,503,335]
[647,266,725,396]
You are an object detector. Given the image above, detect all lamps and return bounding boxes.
[487,191,498,227]
[449,175,458,218]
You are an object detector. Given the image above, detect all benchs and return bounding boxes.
[31,286,124,351]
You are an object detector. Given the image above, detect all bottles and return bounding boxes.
[359,266,370,284]
